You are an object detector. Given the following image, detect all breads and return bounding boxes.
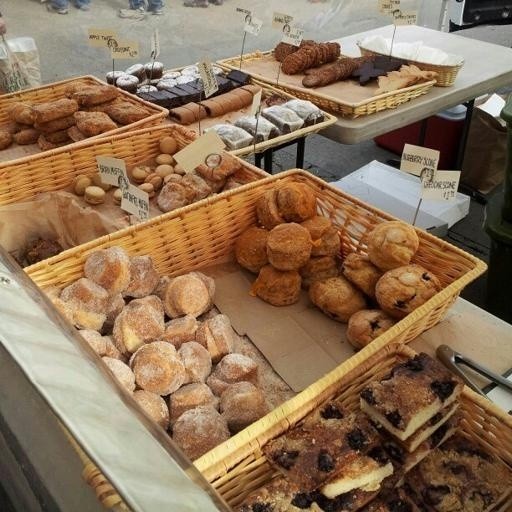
[0,60,512,512]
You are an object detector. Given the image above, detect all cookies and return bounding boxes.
[275,35,439,94]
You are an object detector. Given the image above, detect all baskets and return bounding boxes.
[80,343,511,512]
[20,168,488,481]
[217,49,435,121]
[1,74,170,168]
[141,62,338,164]
[0,39,511,507]
[2,124,273,270]
[358,41,466,87]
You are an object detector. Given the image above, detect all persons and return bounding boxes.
[0,13,9,35]
[183,0,224,8]
[40,0,91,16]
[119,0,166,22]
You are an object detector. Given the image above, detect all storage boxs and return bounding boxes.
[373,90,489,169]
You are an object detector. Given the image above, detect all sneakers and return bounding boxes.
[46,4,87,13]
[184,1,223,8]
[119,7,161,20]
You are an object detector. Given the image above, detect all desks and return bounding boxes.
[254,21,512,205]
